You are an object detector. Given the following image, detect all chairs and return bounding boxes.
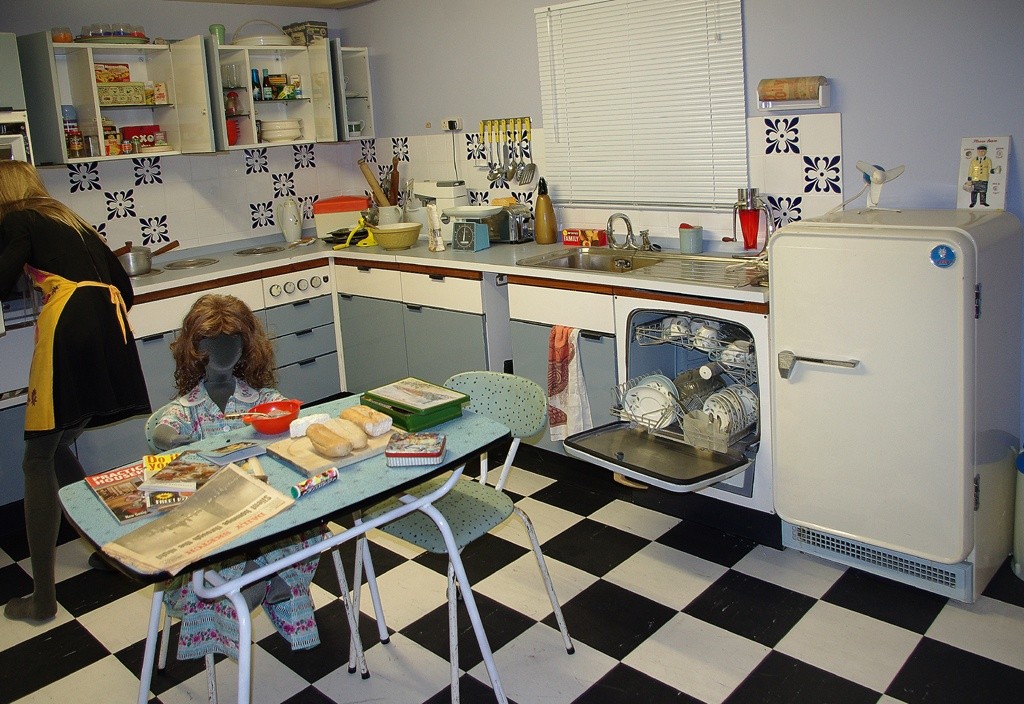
[352,365,576,704]
[146,398,372,704]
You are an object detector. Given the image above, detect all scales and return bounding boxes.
[442,205,503,252]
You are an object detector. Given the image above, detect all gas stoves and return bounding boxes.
[121,236,334,324]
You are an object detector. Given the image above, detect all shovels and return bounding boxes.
[519,117,536,186]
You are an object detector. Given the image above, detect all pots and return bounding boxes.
[116,238,179,276]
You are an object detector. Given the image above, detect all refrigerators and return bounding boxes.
[768,189,1021,572]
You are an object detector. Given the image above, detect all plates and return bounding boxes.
[626,370,758,439]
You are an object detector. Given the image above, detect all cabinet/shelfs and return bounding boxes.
[0,257,340,511]
[208,34,338,148]
[329,250,511,394]
[329,33,375,145]
[2,30,36,170]
[17,32,216,170]
[502,275,623,470]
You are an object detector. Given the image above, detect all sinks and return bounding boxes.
[516,248,668,279]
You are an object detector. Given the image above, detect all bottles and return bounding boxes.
[533,174,558,244]
[263,68,273,100]
[251,68,262,101]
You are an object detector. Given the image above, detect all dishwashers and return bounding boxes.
[562,296,773,510]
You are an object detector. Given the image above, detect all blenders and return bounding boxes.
[408,178,468,243]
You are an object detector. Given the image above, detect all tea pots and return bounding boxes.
[277,196,306,242]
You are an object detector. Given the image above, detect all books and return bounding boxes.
[84,441,267,525]
[956,136,1010,211]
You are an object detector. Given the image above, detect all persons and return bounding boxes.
[967,146,995,208]
[155,293,321,611]
[0,160,134,621]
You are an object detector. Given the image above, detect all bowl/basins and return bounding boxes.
[260,117,305,142]
[241,398,304,434]
[228,118,241,146]
[371,220,423,250]
[660,312,759,370]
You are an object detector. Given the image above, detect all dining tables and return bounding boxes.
[54,385,522,704]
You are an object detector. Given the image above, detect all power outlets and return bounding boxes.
[441,118,463,131]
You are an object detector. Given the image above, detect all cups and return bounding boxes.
[53,26,75,42]
[221,61,242,88]
[348,117,365,137]
[208,23,225,45]
[65,129,87,157]
[132,138,141,153]
[679,224,705,254]
[122,137,134,154]
[80,21,145,38]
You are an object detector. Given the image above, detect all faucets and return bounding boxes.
[603,212,638,252]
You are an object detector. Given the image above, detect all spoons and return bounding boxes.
[224,411,287,420]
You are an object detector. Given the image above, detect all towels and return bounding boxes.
[545,324,593,444]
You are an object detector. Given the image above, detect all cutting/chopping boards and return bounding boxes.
[267,420,410,477]
